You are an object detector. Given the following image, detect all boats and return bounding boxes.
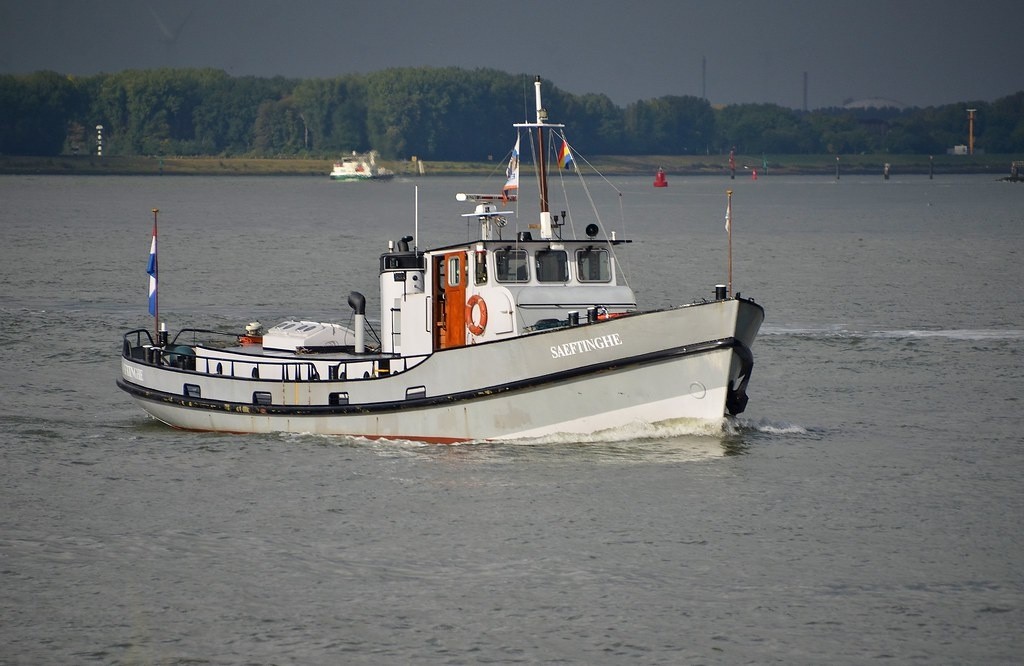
[328,148,396,182]
[116,71,766,441]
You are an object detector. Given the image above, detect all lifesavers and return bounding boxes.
[597,312,628,320]
[465,294,487,336]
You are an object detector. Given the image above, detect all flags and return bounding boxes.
[500,131,520,208]
[557,141,572,171]
[724,204,730,233]
[146,225,157,318]
